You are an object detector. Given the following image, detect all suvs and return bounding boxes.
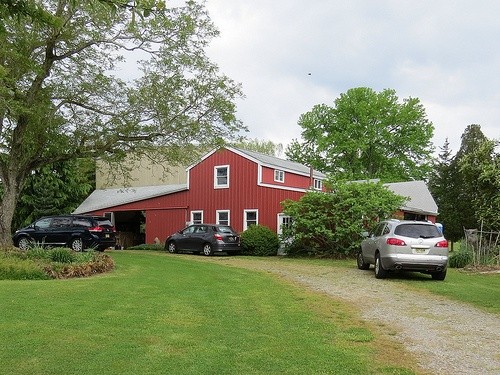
[12,214,116,252]
[357,218,449,280]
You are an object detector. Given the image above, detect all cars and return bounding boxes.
[165,224,241,255]
